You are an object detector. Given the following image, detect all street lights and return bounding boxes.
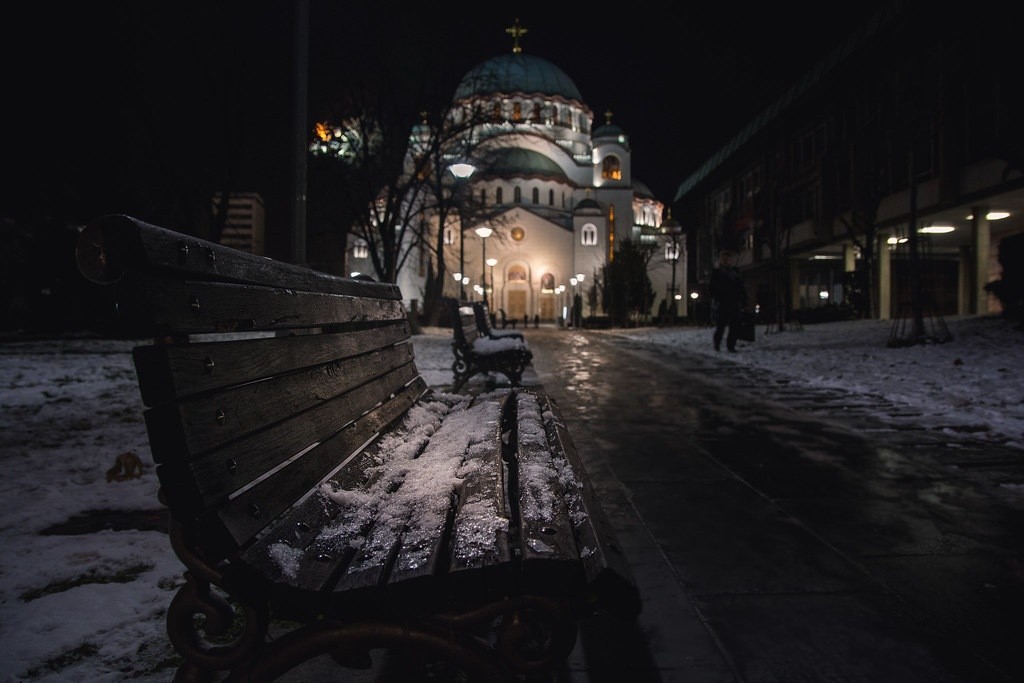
[473,224,495,304]
[447,163,478,304]
[487,259,497,314]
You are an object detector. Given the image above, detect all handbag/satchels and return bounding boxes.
[736,306,755,341]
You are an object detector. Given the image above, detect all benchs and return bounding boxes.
[98,212,642,683]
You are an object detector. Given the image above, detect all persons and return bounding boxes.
[709,249,744,354]
